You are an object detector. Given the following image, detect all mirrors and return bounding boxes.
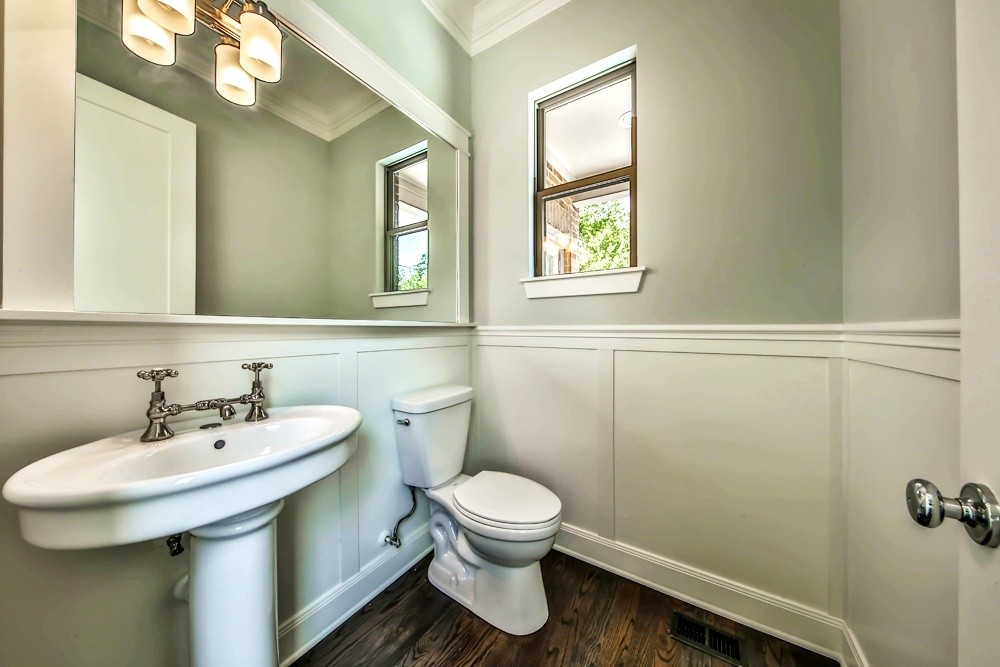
[2,1,461,322]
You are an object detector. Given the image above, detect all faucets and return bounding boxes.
[196,398,238,421]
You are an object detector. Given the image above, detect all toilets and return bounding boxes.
[391,384,563,636]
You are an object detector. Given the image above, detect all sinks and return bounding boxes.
[1,404,363,549]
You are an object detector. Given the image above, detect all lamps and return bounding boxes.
[121,0,284,106]
